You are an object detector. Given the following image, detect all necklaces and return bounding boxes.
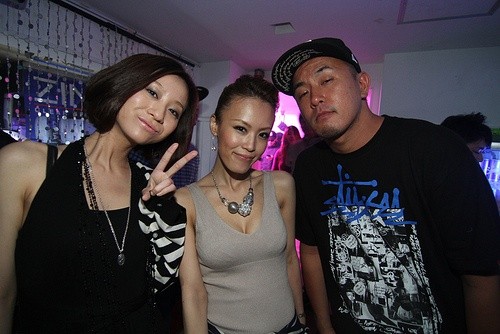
[209,170,254,217]
[78,134,162,334]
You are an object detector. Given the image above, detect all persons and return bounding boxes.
[253,111,324,177]
[288,45,500,334]
[437,111,493,163]
[0,53,199,334]
[171,75,312,334]
[128,91,199,198]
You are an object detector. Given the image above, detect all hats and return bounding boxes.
[271,37,360,97]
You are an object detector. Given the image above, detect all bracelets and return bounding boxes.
[297,313,306,318]
